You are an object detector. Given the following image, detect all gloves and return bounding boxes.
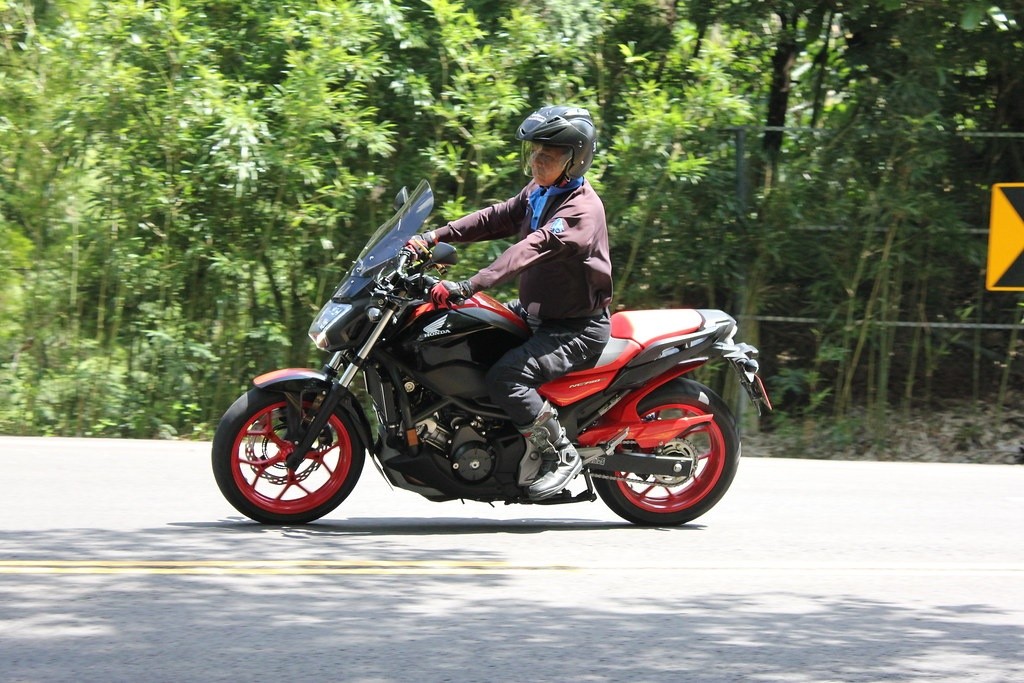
[431,279,472,311]
[402,231,438,261]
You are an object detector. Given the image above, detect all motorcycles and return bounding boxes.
[209,175,776,530]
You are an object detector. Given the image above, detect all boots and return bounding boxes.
[516,397,582,501]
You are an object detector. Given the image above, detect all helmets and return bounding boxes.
[515,105,597,180]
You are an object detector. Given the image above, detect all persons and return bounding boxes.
[405,105,614,501]
